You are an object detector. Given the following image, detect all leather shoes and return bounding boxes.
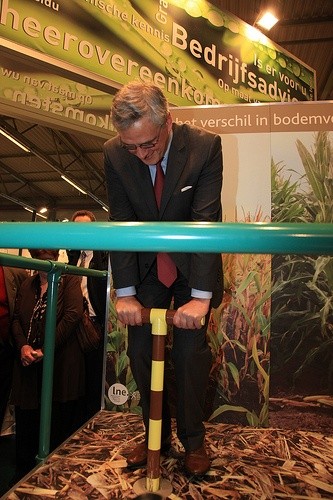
[126,441,170,467]
[184,440,211,479]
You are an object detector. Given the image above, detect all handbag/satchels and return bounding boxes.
[63,274,100,353]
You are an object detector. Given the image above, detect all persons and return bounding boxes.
[0,210,108,476]
[104,81,222,478]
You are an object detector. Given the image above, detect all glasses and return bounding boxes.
[119,119,166,151]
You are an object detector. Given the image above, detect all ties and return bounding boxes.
[78,251,87,284]
[153,156,179,290]
